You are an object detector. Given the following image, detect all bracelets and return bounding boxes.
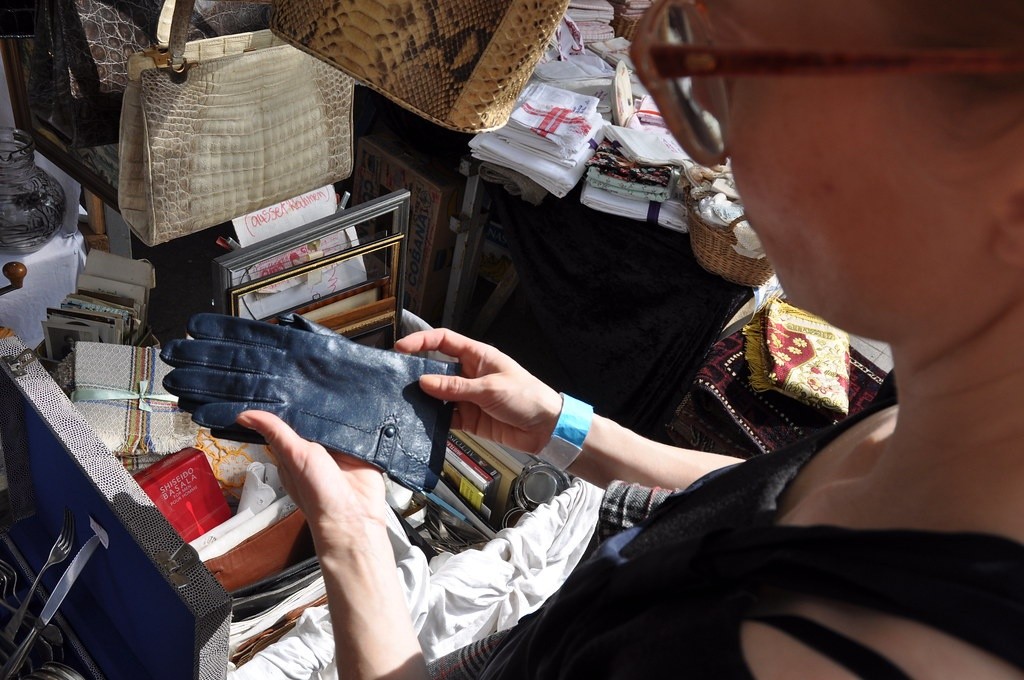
[534,391,594,473]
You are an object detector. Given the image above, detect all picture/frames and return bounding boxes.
[205,187,405,357]
[1,36,145,215]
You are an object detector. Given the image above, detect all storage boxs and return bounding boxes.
[348,131,479,321]
[473,218,523,287]
[0,335,231,680]
[33,328,164,378]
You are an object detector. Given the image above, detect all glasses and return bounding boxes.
[630,2,1023,168]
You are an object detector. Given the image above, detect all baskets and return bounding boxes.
[686,182,776,287]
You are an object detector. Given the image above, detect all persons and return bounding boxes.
[62,336,76,358]
[233,0,1024,678]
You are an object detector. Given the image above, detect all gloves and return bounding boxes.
[159,312,463,494]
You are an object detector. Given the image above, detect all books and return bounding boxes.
[221,422,545,671]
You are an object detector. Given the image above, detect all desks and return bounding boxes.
[0,228,86,356]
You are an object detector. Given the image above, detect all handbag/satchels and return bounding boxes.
[270,0,570,134]
[596,479,685,546]
[118,1,354,245]
